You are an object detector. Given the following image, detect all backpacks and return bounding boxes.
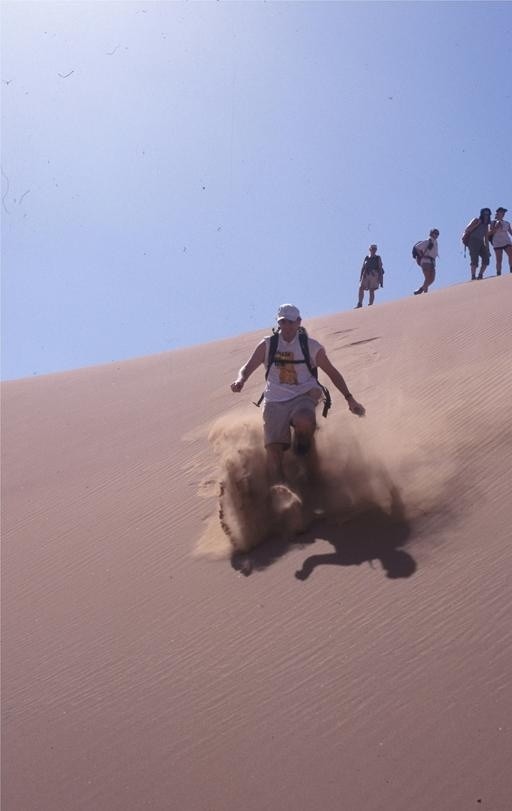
[462,217,480,246]
[413,239,433,264]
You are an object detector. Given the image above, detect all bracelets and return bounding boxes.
[345,394,352,400]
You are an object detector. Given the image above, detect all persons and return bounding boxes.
[230,304,366,478]
[353,244,384,309]
[413,229,440,296]
[461,208,492,282]
[487,208,511,276]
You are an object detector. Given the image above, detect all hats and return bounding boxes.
[481,208,490,215]
[276,304,300,322]
[369,245,377,250]
[496,208,507,213]
[430,228,438,236]
